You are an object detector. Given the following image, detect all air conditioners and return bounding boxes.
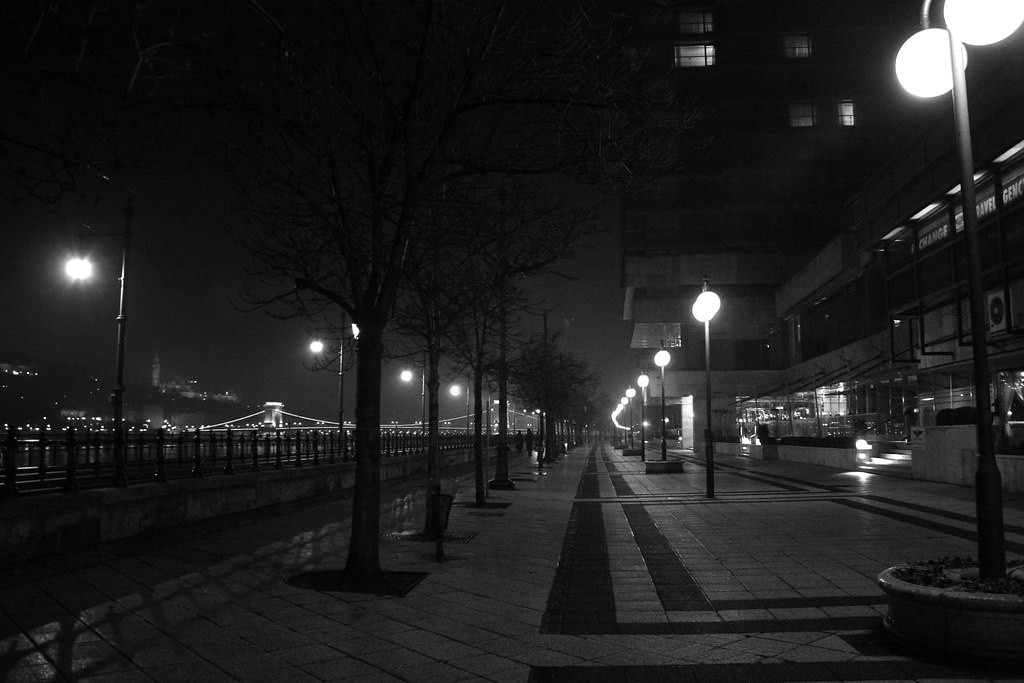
[987,286,1024,333]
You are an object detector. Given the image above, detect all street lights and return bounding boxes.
[653,339,671,462]
[310,336,343,448]
[692,279,722,500]
[449,384,472,436]
[637,369,650,463]
[611,396,628,449]
[401,368,426,432]
[626,385,636,450]
[62,245,130,487]
[892,1,1024,592]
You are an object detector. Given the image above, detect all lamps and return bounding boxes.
[921,390,935,401]
